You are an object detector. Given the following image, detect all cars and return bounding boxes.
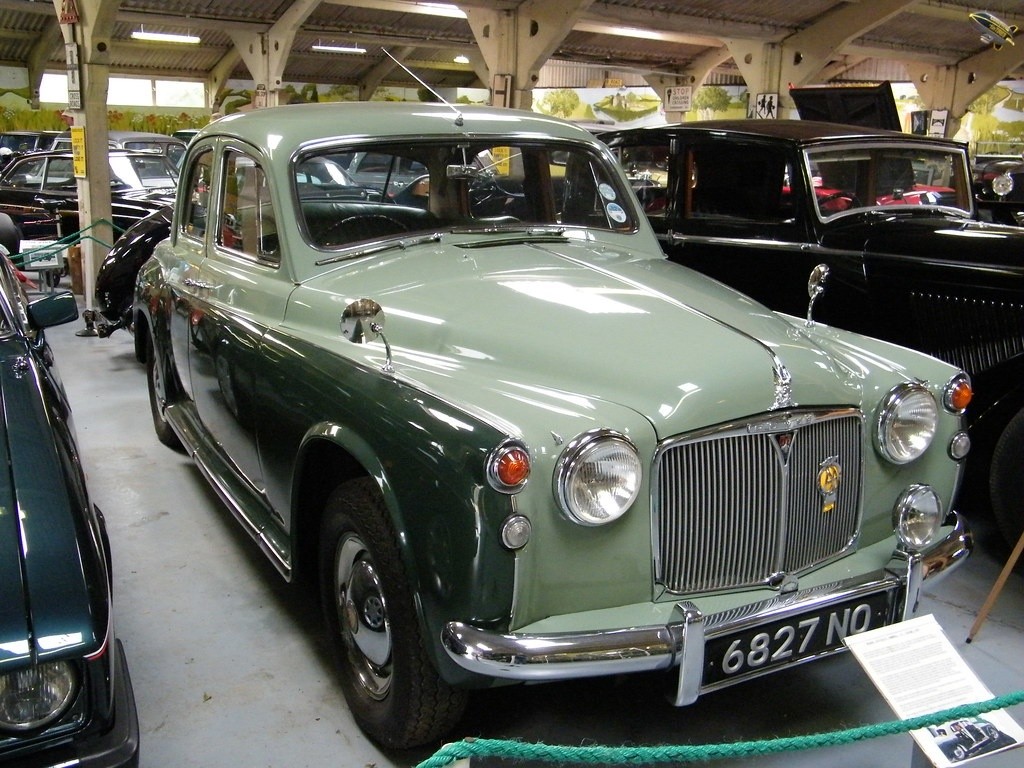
[134,101,970,752]
[0,129,508,336]
[528,118,1024,577]
[1,245,138,768]
[0,214,139,768]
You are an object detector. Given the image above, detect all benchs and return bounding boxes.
[234,192,363,255]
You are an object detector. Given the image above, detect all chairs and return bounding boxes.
[295,200,436,245]
